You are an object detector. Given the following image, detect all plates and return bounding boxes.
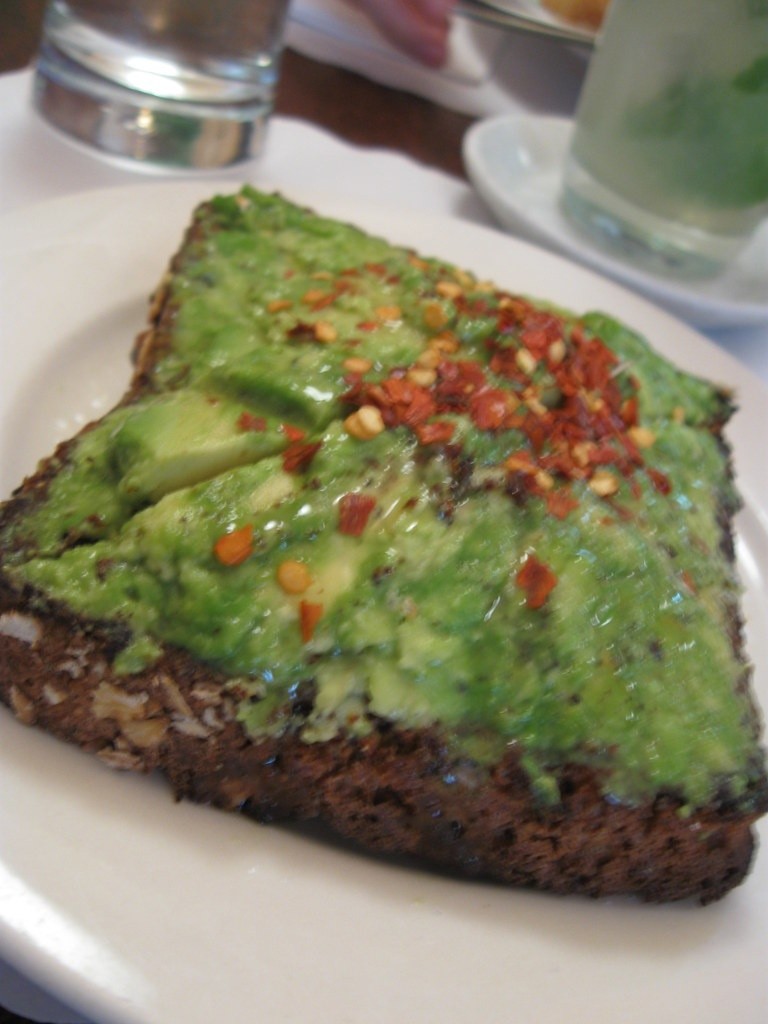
[460,110,768,333]
[0,168,765,1024]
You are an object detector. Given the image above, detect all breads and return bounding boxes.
[1,189,768,908]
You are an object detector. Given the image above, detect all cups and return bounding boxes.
[564,0,768,285]
[25,0,287,168]
